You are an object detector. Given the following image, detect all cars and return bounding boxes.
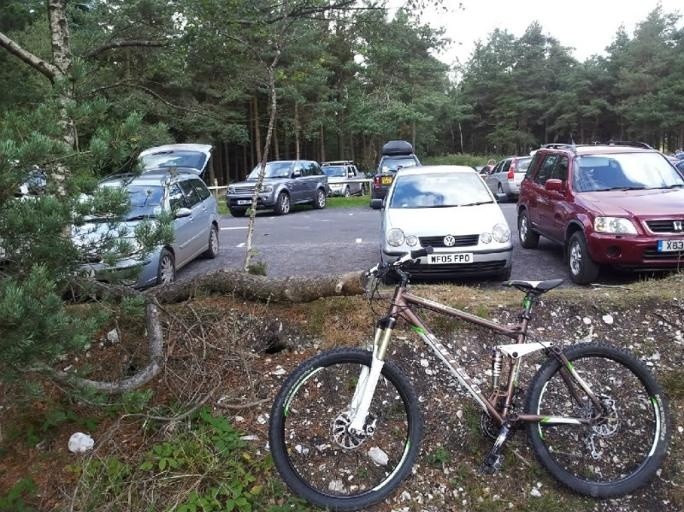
[474,143,577,202]
[60,141,220,293]
[378,164,513,282]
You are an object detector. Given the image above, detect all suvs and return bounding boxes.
[517,139,684,285]
[370,138,423,200]
[223,158,330,215]
[318,160,368,199]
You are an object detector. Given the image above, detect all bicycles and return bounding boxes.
[264,243,672,511]
